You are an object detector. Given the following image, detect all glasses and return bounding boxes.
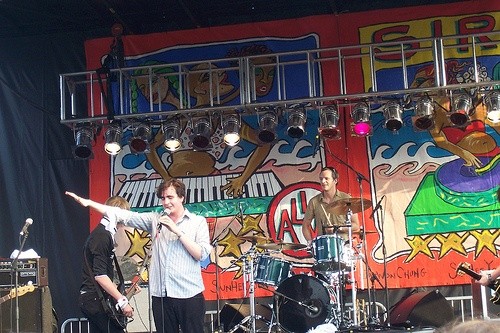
[324,167,335,174]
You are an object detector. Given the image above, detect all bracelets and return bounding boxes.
[177,231,185,240]
[115,295,129,311]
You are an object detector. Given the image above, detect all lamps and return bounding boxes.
[73,129,95,161]
[382,99,403,131]
[483,91,500,123]
[414,96,434,131]
[220,114,241,147]
[350,103,372,136]
[258,110,278,143]
[318,106,340,140]
[286,108,307,139]
[449,94,472,125]
[161,121,183,152]
[129,122,152,153]
[103,125,122,155]
[191,115,213,151]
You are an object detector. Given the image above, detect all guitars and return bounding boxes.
[455,262,500,305]
[105,269,149,330]
[0,284,35,305]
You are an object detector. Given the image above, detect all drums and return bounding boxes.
[314,248,355,274]
[312,236,345,272]
[273,274,339,333]
[254,255,294,285]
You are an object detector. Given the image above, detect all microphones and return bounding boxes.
[19,218,33,238]
[308,306,319,313]
[370,196,384,218]
[158,208,171,225]
[312,135,319,158]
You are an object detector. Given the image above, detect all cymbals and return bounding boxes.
[240,236,271,242]
[325,198,373,215]
[256,242,307,251]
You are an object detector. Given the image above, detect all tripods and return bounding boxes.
[226,240,281,333]
[320,145,406,333]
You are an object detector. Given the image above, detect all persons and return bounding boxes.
[432,312,500,333]
[65,178,213,333]
[77,196,142,333]
[475,187,500,287]
[302,167,360,329]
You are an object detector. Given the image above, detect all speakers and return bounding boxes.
[0,258,53,333]
[208,302,272,333]
[387,288,456,329]
[123,285,157,333]
